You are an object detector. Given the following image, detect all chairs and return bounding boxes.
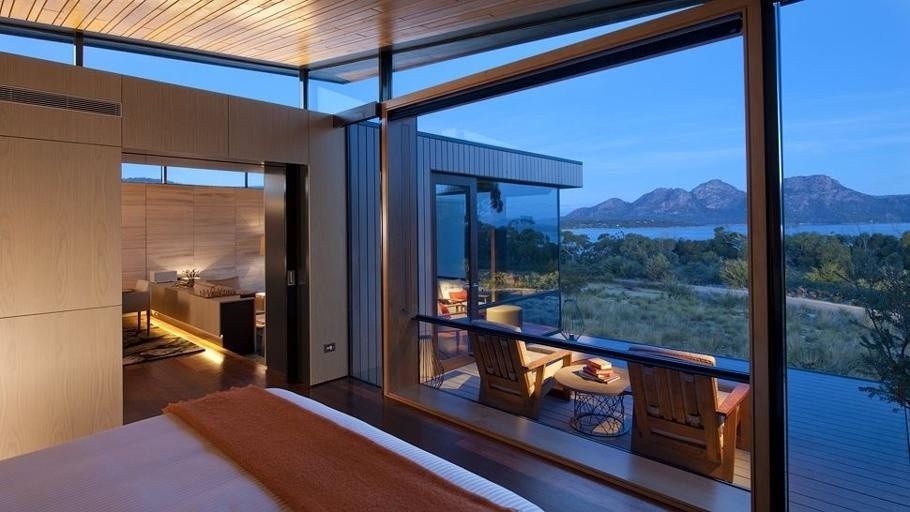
[621,341,755,483]
[465,318,572,424]
[121,278,152,337]
[436,279,489,322]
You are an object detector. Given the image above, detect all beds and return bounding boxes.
[1,377,544,511]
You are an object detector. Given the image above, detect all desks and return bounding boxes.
[550,363,633,438]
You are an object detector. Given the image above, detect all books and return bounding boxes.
[582,358,621,384]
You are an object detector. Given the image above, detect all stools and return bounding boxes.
[486,304,525,330]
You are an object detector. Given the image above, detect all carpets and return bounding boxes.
[122,316,204,369]
